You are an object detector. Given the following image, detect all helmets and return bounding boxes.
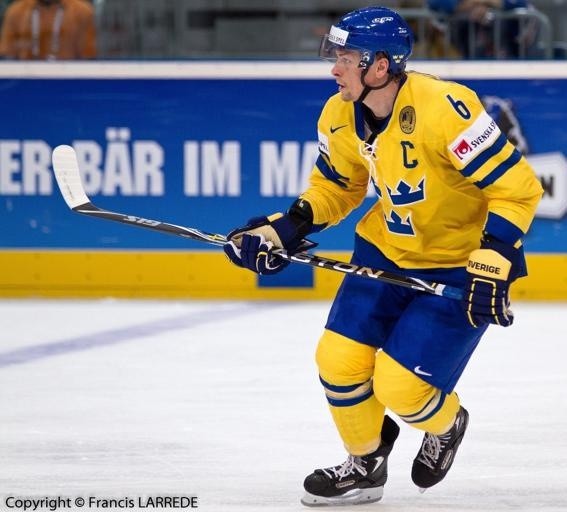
[319,6,415,75]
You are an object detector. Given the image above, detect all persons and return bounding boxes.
[224,6,545,497]
[0,0,96,59]
[428,0,540,58]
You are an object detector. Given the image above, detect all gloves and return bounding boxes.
[462,248,514,329]
[223,212,317,276]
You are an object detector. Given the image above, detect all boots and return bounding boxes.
[411,405,470,488]
[304,415,400,497]
